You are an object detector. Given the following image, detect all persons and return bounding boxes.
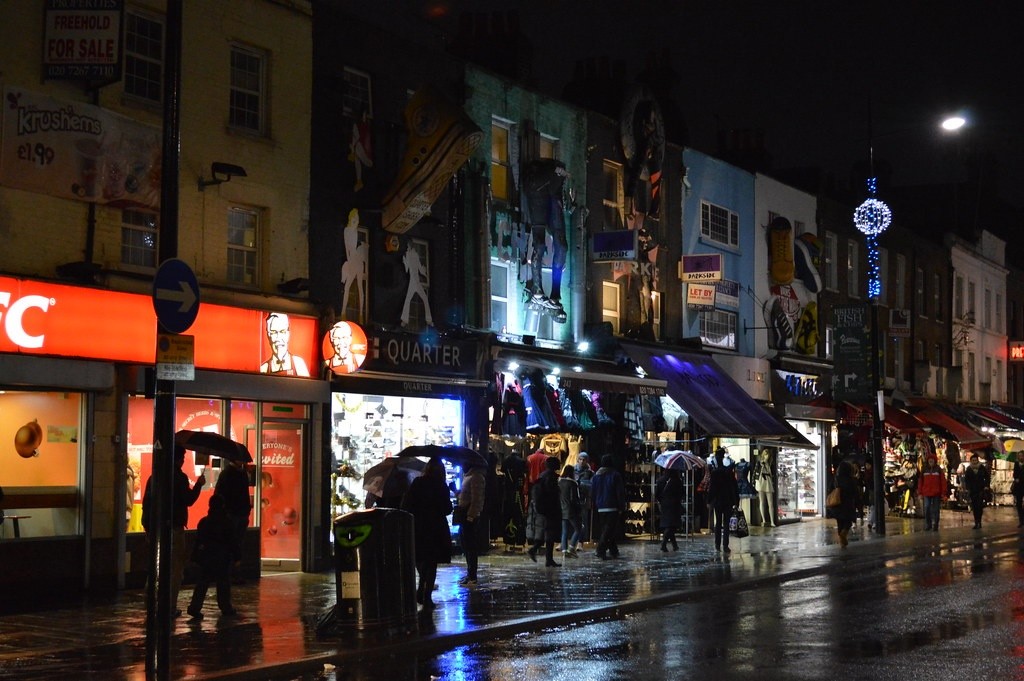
[188,459,252,619]
[527,457,562,567]
[965,454,991,531]
[698,450,776,533]
[559,450,592,558]
[1012,450,1024,528]
[863,460,876,531]
[402,457,452,607]
[591,454,623,557]
[501,449,549,545]
[522,157,568,323]
[365,468,409,507]
[918,455,946,530]
[711,447,738,554]
[833,462,856,550]
[655,469,685,553]
[144,446,205,616]
[450,461,486,586]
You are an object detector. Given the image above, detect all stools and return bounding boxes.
[4,515,32,537]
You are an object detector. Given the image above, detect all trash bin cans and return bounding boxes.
[333,508,418,637]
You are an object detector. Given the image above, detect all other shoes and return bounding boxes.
[461,578,477,586]
[595,549,612,560]
[546,559,562,567]
[839,529,848,548]
[187,608,202,619]
[171,609,181,621]
[223,609,236,616]
[567,547,578,558]
[925,524,932,530]
[933,526,938,531]
[724,547,730,552]
[561,552,570,557]
[972,523,981,528]
[611,549,619,557]
[416,591,433,608]
[660,546,669,552]
[673,546,679,551]
[716,548,721,552]
[528,548,537,563]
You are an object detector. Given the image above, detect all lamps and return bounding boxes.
[198,162,247,192]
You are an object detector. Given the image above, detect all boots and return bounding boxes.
[576,542,583,550]
[555,543,562,551]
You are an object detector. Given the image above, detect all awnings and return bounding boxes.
[621,341,820,450]
[498,349,668,396]
[844,395,1024,447]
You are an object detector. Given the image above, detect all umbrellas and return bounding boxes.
[363,459,429,481]
[176,429,253,474]
[445,444,490,465]
[397,445,460,457]
[655,449,705,471]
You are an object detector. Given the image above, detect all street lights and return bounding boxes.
[850,98,973,534]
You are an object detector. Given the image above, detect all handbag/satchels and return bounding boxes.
[729,507,749,538]
[826,476,841,507]
